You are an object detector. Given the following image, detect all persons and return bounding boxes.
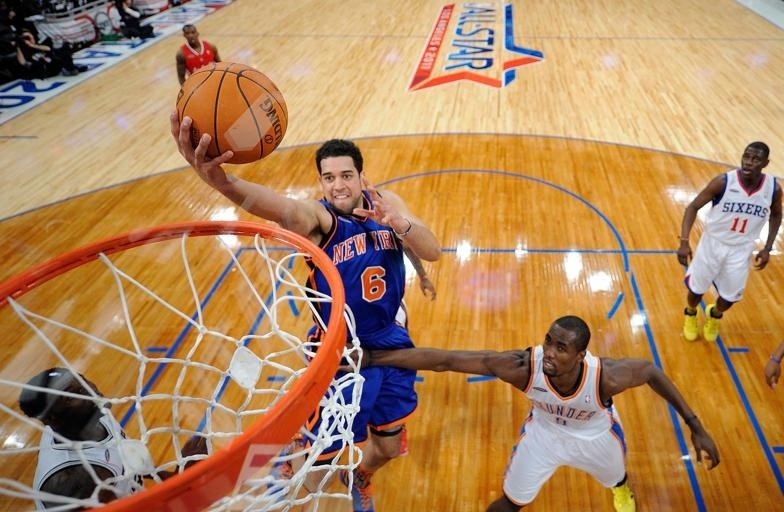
[19,367,207,512]
[395,242,437,330]
[763,344,783,389]
[676,142,783,342]
[176,24,223,86]
[0,0,156,80]
[338,316,721,512]
[172,111,442,512]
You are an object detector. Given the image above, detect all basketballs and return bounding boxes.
[175,62,288,165]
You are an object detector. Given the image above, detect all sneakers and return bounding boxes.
[61,64,87,76]
[267,445,293,505]
[610,479,636,511]
[683,307,699,341]
[703,304,722,343]
[338,469,375,512]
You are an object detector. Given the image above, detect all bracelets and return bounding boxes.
[686,416,696,425]
[392,218,412,237]
[678,236,689,241]
[765,245,772,252]
[365,350,372,369]
[769,353,781,364]
[421,274,428,281]
